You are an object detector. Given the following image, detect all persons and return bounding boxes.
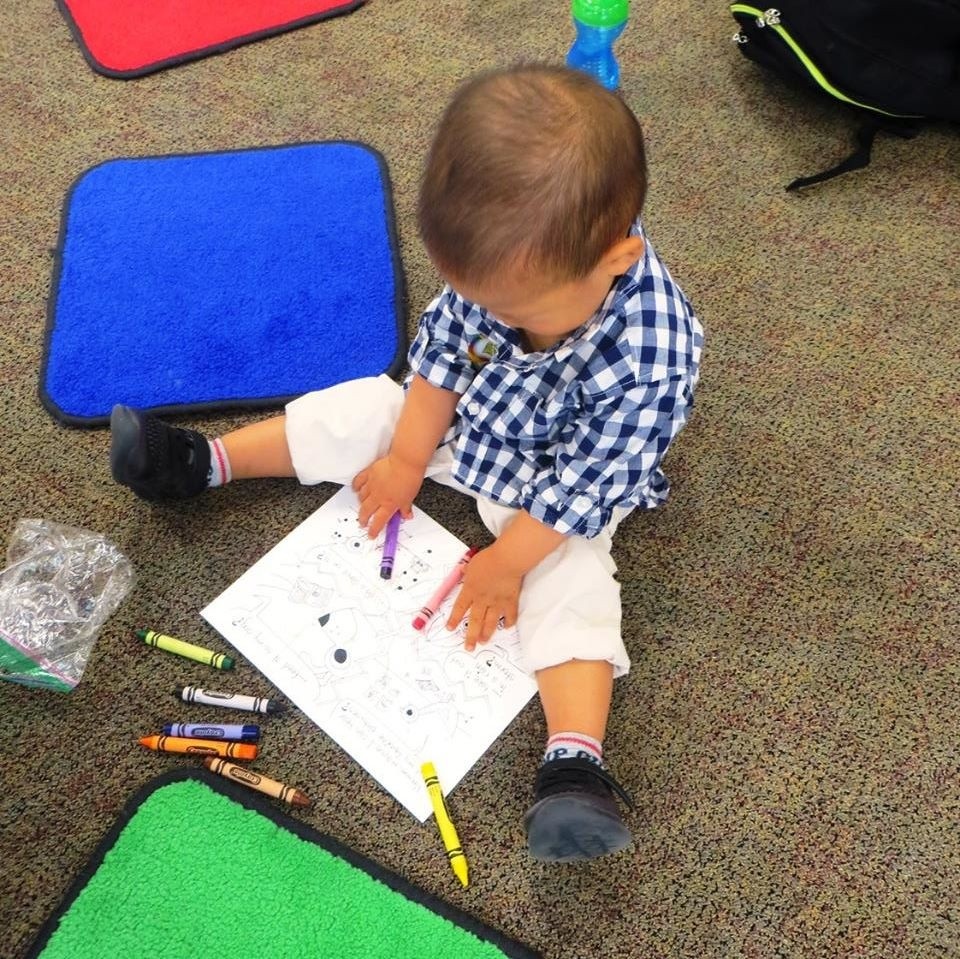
[111,61,706,861]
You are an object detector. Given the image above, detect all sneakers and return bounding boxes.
[524,760,634,862]
[110,401,212,502]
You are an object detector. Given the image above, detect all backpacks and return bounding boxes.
[731,1,960,130]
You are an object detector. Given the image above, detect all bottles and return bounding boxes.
[566,0,628,92]
